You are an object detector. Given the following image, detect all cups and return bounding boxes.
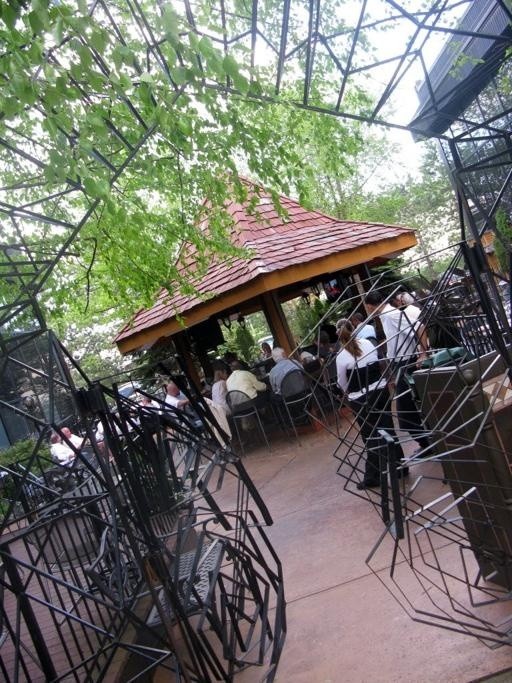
[259,366,266,377]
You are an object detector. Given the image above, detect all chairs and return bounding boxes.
[87,503,238,670]
[278,369,328,448]
[224,390,283,457]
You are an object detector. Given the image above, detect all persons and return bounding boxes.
[177,375,196,414]
[224,352,250,370]
[396,292,422,321]
[211,366,256,431]
[227,362,267,414]
[351,313,376,340]
[335,319,409,490]
[313,331,336,363]
[61,427,90,451]
[268,346,305,406]
[364,290,433,459]
[50,433,75,461]
[164,384,206,428]
[261,342,272,361]
[142,395,158,417]
